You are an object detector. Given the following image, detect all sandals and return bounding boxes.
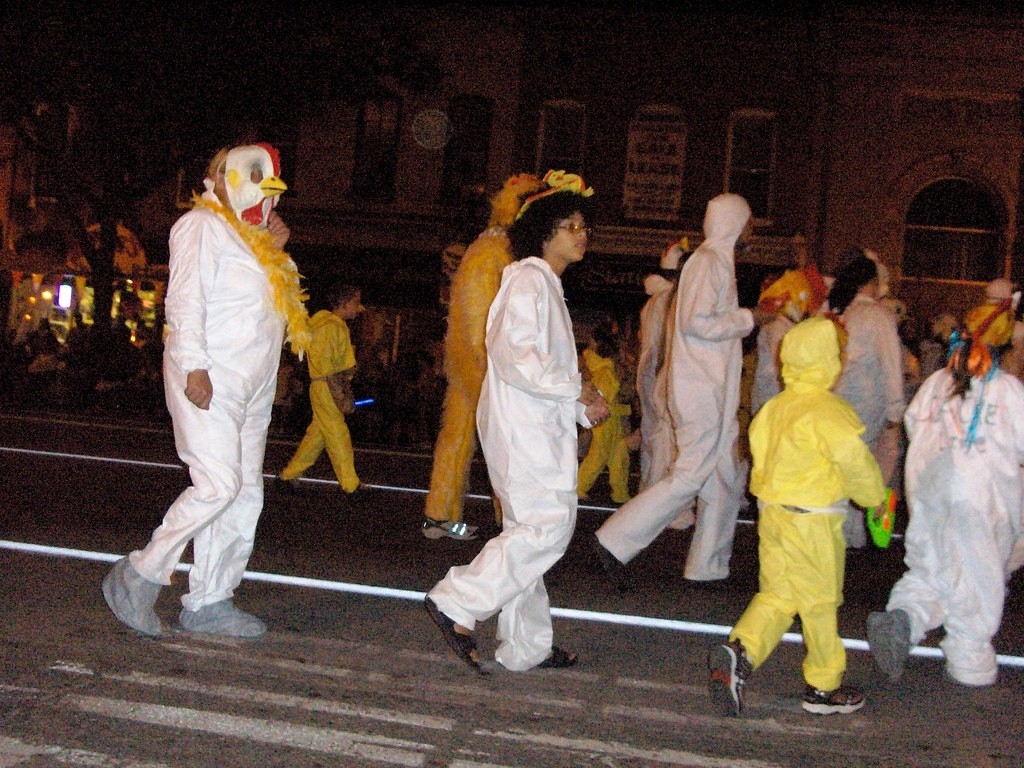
[424,598,489,675]
[537,646,578,668]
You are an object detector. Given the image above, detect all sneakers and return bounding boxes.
[707,642,751,718]
[801,685,865,715]
[421,517,479,540]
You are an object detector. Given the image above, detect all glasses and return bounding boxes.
[554,223,589,235]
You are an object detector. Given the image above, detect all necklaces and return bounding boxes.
[187,190,311,354]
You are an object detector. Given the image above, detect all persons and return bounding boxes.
[708,309,897,717]
[101,140,312,638]
[575,191,779,606]
[0,174,1024,687]
[423,170,613,676]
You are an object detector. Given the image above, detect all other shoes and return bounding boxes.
[274,475,308,497]
[593,538,631,592]
[685,576,748,594]
[346,484,387,503]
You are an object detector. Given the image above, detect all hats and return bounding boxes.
[960,306,1011,346]
